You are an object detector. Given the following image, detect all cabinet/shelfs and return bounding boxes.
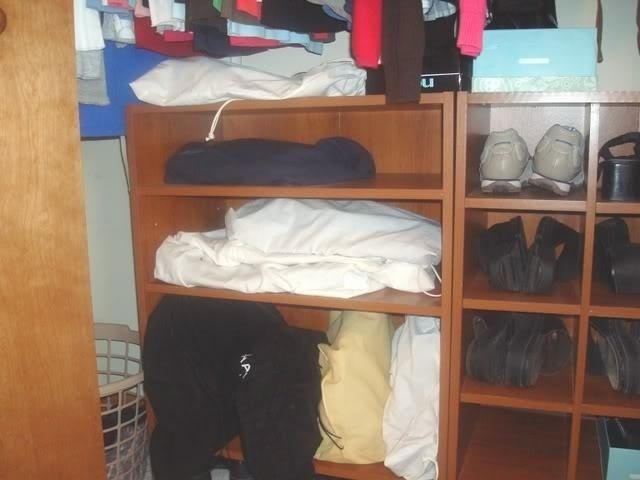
[123,88,456,480]
[448,90,640,479]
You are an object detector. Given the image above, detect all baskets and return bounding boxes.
[92,322,145,479]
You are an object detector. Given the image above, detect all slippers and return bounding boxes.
[590,318,631,396]
[478,217,525,293]
[523,214,581,295]
[464,313,517,383]
[503,312,571,387]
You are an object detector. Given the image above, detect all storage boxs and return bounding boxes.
[471,26,600,93]
[595,415,640,480]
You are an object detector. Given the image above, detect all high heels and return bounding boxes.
[598,217,640,294]
[599,132,640,201]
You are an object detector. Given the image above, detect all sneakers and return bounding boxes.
[528,122,586,195]
[479,127,532,194]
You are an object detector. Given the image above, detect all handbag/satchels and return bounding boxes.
[383,315,440,480]
[313,310,395,465]
[240,324,329,480]
[144,293,283,479]
[129,56,368,143]
